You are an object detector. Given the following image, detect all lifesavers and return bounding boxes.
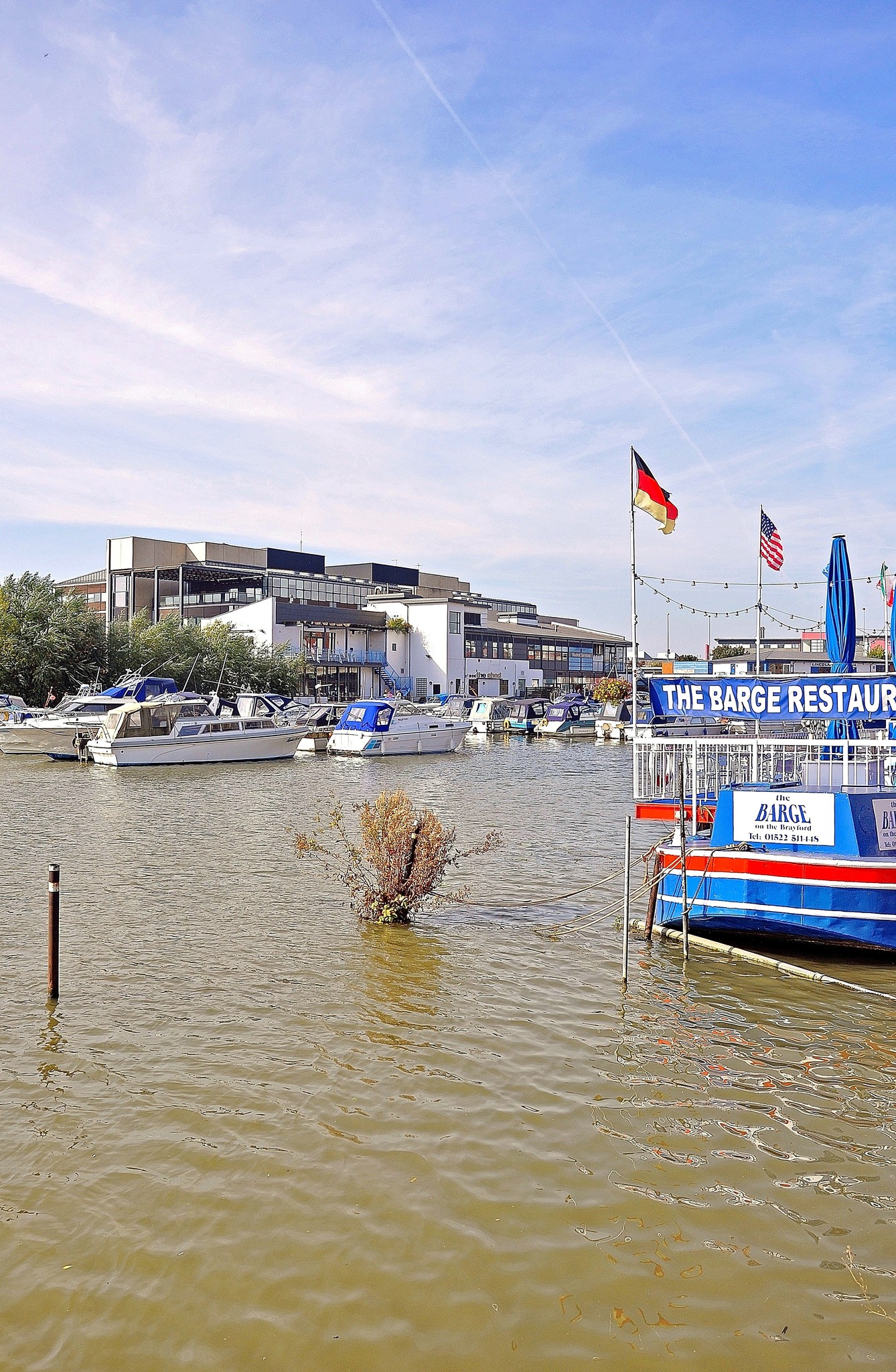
[0,708,9,722]
[649,744,675,786]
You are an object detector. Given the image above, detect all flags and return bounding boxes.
[631,448,678,535]
[759,509,784,572]
[876,560,896,608]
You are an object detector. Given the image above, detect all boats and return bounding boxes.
[0,647,828,768]
